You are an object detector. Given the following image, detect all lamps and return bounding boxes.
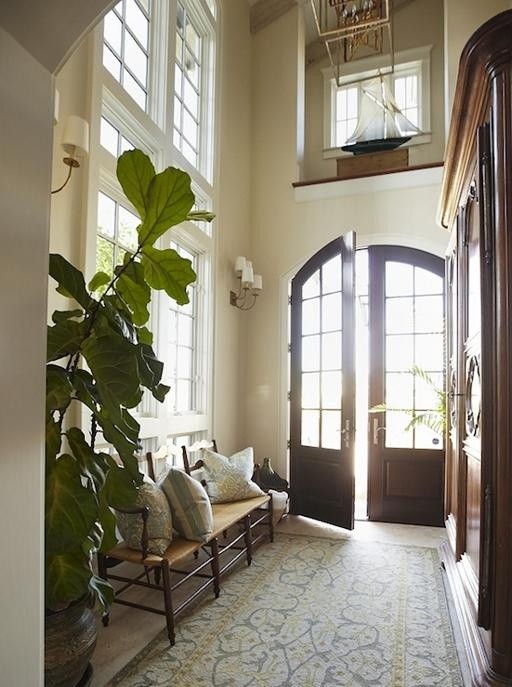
[228,256,264,314]
[50,114,90,195]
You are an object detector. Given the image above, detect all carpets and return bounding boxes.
[109,527,468,687]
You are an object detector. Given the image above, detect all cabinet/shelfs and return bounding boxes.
[436,5,512,687]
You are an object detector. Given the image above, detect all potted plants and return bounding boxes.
[44,147,216,686]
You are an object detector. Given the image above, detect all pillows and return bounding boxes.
[114,442,264,556]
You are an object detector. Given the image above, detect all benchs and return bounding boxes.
[92,433,275,647]
